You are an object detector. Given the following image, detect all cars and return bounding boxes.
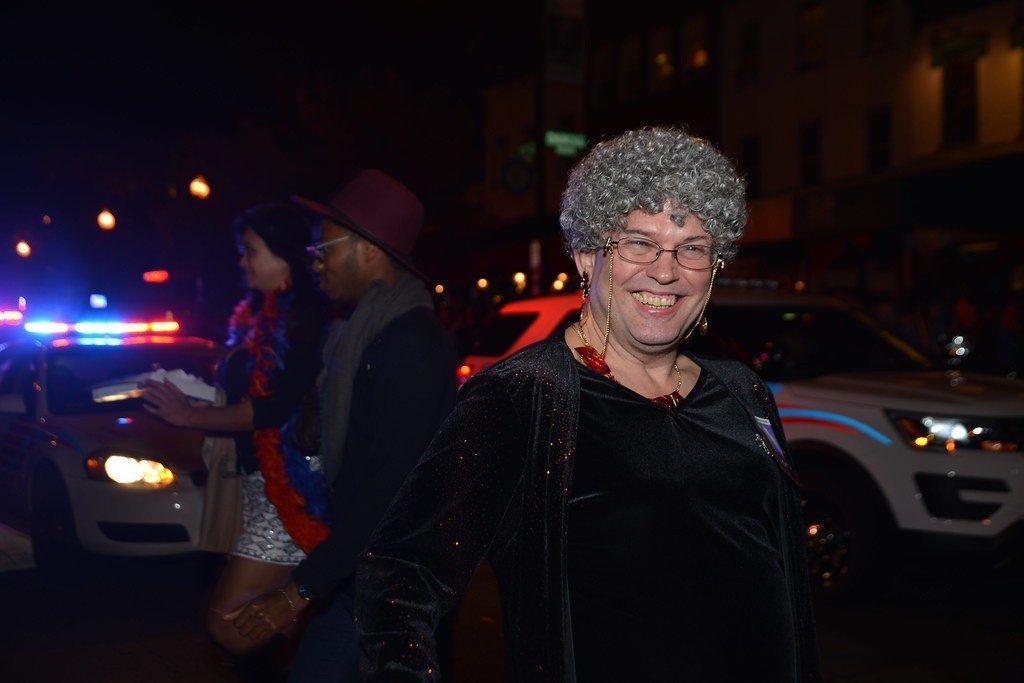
[454,278,1024,600]
[0,312,231,605]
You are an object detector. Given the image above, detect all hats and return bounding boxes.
[287,170,435,282]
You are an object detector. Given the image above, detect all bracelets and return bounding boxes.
[277,589,296,622]
[298,586,313,600]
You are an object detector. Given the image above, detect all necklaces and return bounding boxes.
[572,323,683,408]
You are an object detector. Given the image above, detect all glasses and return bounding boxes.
[599,237,721,271]
[307,232,359,263]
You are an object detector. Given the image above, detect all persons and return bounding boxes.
[142,207,338,683]
[223,170,457,682]
[355,128,825,683]
[820,243,1024,378]
